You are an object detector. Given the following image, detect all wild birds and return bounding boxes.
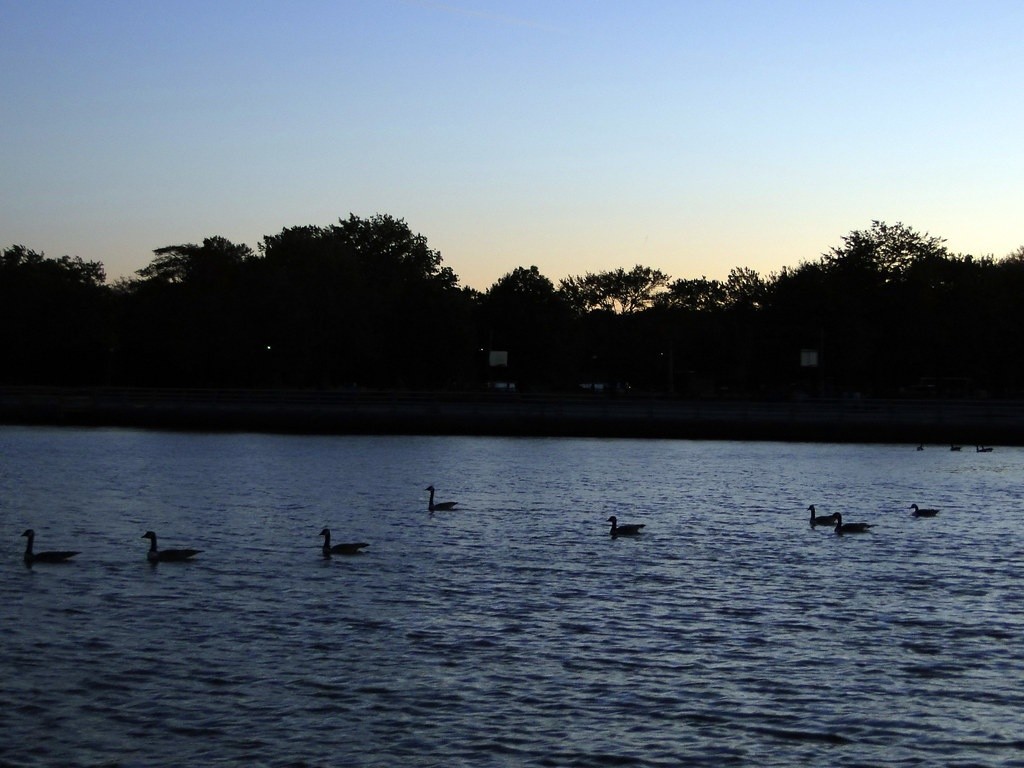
[949,443,962,452]
[806,504,880,535]
[318,528,368,556]
[908,503,943,518]
[425,485,459,512]
[916,444,925,451]
[606,516,646,538]
[140,530,206,562]
[974,444,994,452]
[18,528,83,568]
